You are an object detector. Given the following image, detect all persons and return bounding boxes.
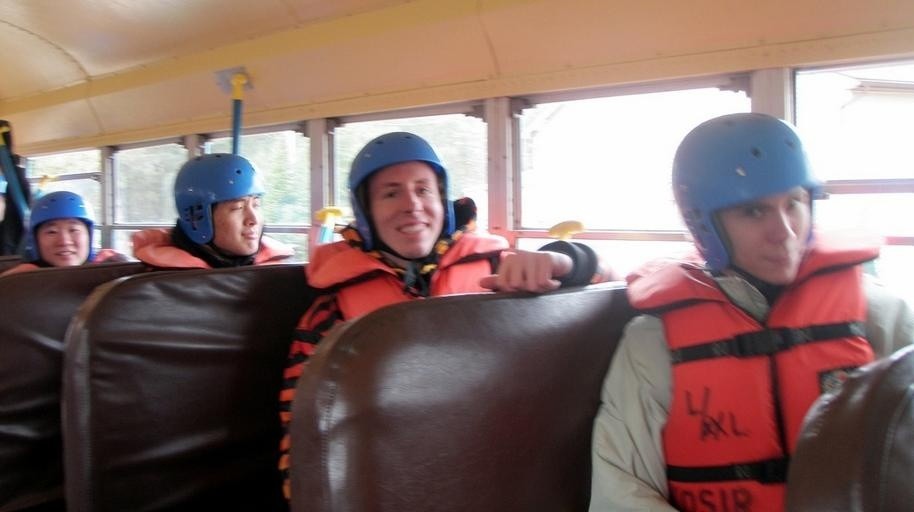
[0,191,127,276]
[277,132,622,499]
[589,112,914,512]
[131,154,296,270]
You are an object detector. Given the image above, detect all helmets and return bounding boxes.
[345,132,457,248]
[671,113,831,275]
[28,191,94,263]
[173,153,266,246]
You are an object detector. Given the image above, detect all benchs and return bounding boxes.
[789,333,914,508]
[0,258,147,509]
[60,260,309,510]
[286,277,630,511]
